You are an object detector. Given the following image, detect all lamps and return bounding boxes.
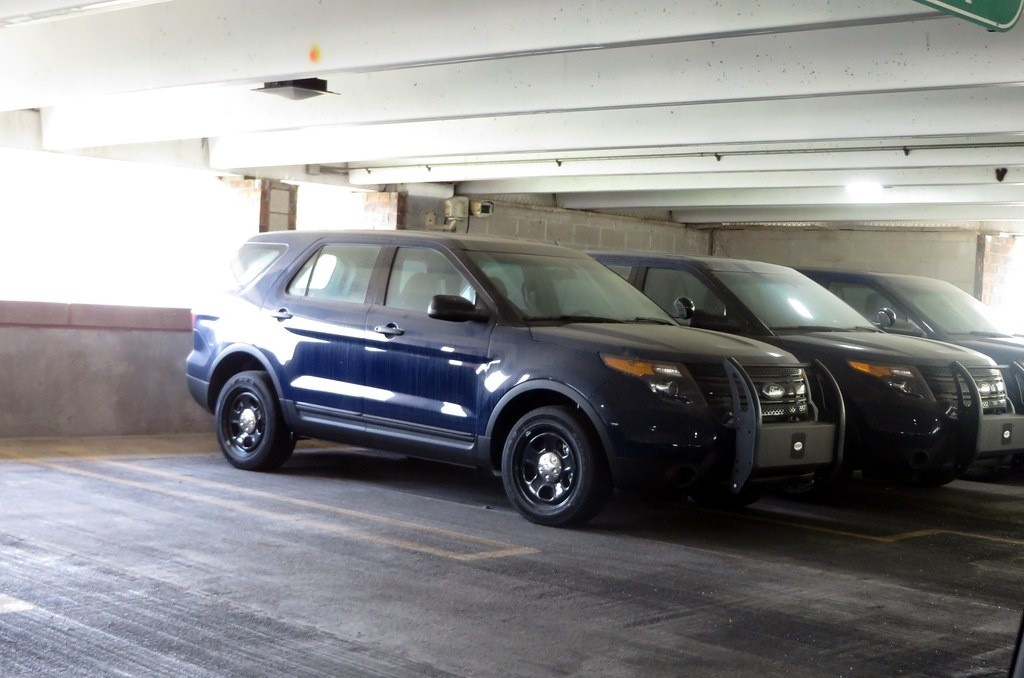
[253,76,342,101]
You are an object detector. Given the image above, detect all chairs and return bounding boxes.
[397,274,436,312]
[474,278,514,311]
[865,292,902,324]
[644,270,690,314]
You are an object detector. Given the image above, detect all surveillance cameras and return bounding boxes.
[468,199,494,217]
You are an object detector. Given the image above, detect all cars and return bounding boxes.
[415,249,1024,509]
[184,227,838,527]
[694,264,1024,450]
